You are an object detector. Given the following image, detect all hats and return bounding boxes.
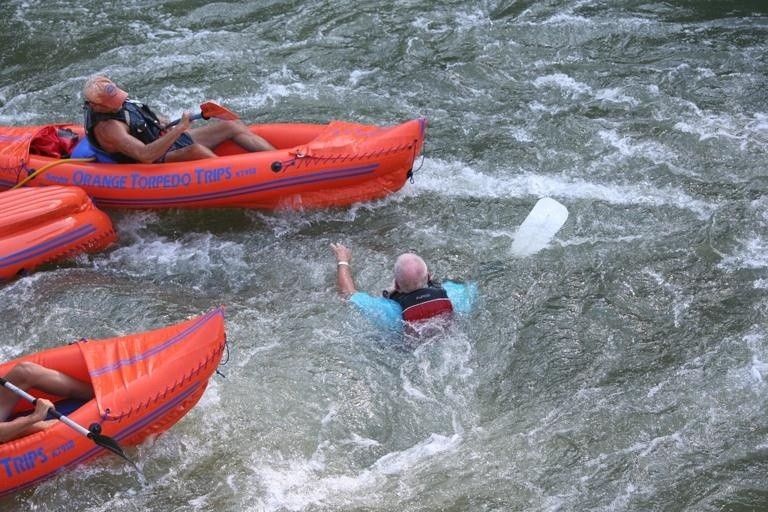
[83,77,129,110]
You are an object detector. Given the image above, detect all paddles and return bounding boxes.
[160,101,244,126]
[0,375,144,475]
[506,195,570,265]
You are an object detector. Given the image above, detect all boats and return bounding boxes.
[0,304,226,501]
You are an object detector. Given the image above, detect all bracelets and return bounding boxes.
[336,260,352,269]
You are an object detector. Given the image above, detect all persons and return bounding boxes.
[81,75,276,164]
[327,239,481,338]
[0,360,95,445]
